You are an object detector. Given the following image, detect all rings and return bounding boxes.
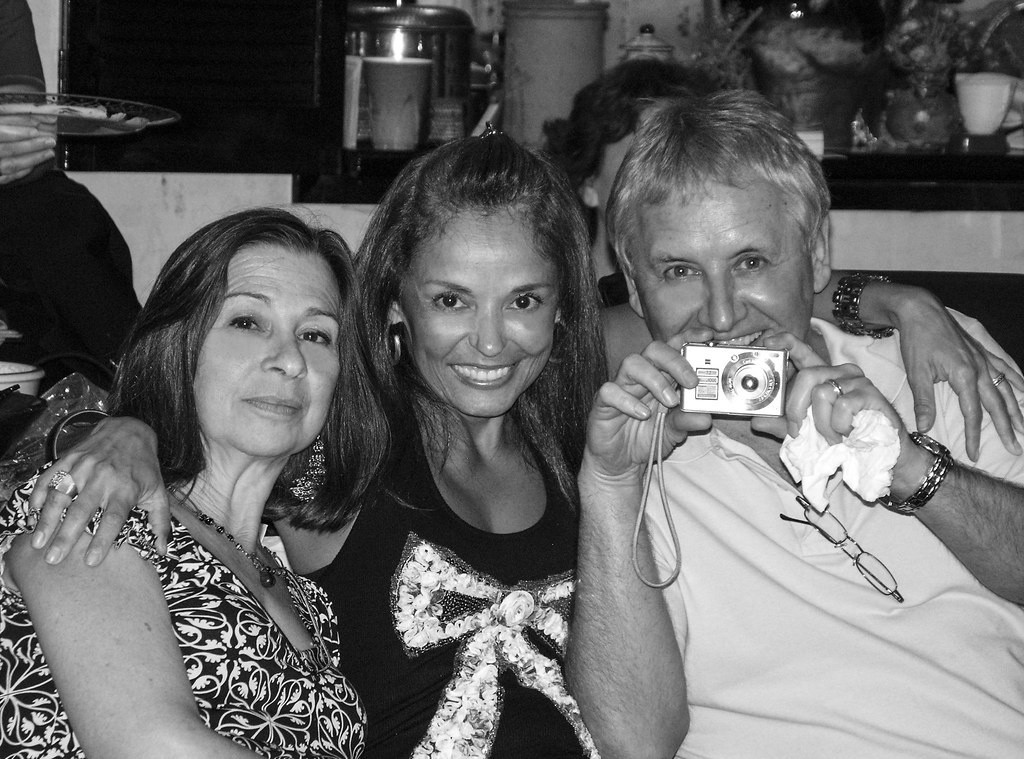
[992,373,1007,387]
[48,470,80,502]
[824,378,843,397]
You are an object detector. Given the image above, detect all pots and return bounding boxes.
[343,4,474,98]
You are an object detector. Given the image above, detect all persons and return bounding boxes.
[1,0,1023,759]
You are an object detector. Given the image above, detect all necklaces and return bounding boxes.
[170,480,284,586]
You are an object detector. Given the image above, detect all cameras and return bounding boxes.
[680,342,790,418]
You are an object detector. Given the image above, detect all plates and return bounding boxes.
[0,90,183,135]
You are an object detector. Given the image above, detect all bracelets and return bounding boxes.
[832,270,897,340]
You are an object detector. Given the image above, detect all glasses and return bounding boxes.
[779,496,905,604]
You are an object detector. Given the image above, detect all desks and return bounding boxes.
[291,185,1024,276]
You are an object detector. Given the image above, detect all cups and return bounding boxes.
[955,72,1019,135]
[0,361,47,396]
[362,57,433,150]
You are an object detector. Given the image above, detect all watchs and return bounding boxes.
[883,429,955,517]
